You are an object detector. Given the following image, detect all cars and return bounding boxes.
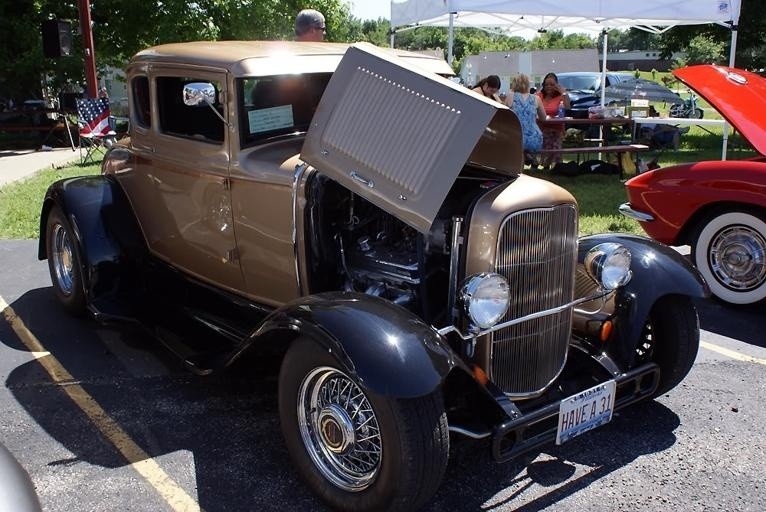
[552,72,622,131]
[615,63,766,319]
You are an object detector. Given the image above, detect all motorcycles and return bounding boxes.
[668,88,705,121]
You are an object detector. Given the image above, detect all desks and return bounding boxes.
[538,118,633,163]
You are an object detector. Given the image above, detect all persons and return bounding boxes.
[292,8,325,44]
[533,73,572,169]
[471,75,502,104]
[504,73,545,164]
[639,124,690,147]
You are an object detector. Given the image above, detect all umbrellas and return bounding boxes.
[595,75,685,111]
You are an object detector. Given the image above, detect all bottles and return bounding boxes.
[558,100,564,119]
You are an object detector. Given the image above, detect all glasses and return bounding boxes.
[317,25,326,31]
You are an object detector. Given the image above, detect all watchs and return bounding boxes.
[561,92,568,97]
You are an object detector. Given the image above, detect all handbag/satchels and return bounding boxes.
[655,124,681,148]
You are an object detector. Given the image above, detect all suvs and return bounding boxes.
[35,37,707,512]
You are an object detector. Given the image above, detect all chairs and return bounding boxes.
[75,97,130,167]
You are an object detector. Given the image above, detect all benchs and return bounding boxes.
[539,144,649,181]
